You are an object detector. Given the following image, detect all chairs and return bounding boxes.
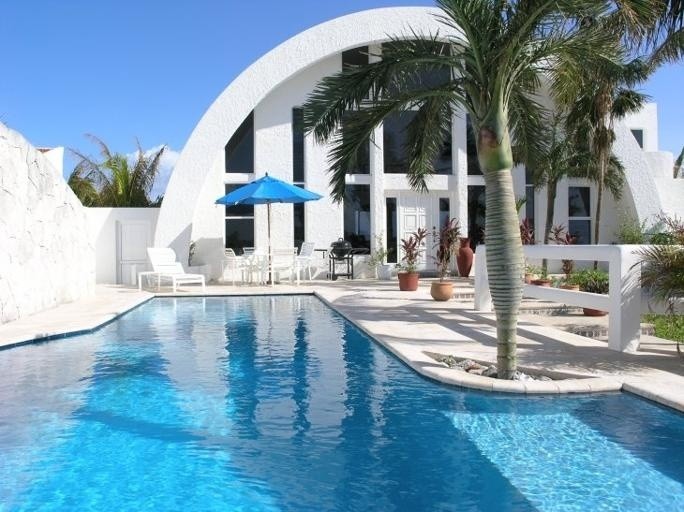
[138,247,204,291]
[221,241,316,287]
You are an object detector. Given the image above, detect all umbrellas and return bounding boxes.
[214,171,324,285]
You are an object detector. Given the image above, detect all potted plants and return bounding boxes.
[521,220,610,317]
[369,214,461,302]
